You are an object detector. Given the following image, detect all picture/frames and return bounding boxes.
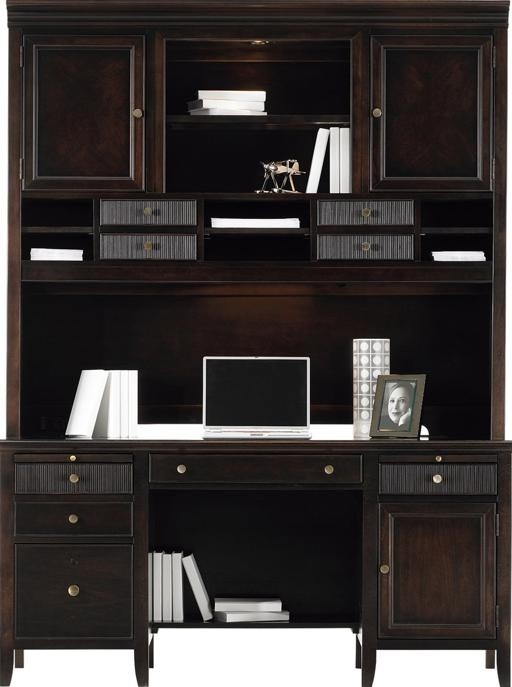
[369,373,424,438]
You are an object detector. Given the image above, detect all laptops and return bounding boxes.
[201,356,313,440]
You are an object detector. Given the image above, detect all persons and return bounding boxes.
[387,382,413,428]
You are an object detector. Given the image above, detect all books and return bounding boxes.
[339,126,351,193]
[32,255,83,262]
[29,247,84,255]
[330,126,340,194]
[434,257,486,262]
[430,250,484,257]
[197,89,267,101]
[200,98,265,112]
[148,551,289,624]
[63,369,138,440]
[304,127,330,194]
[191,108,267,116]
[210,216,301,229]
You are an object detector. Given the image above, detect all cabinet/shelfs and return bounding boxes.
[0,0,511,687]
